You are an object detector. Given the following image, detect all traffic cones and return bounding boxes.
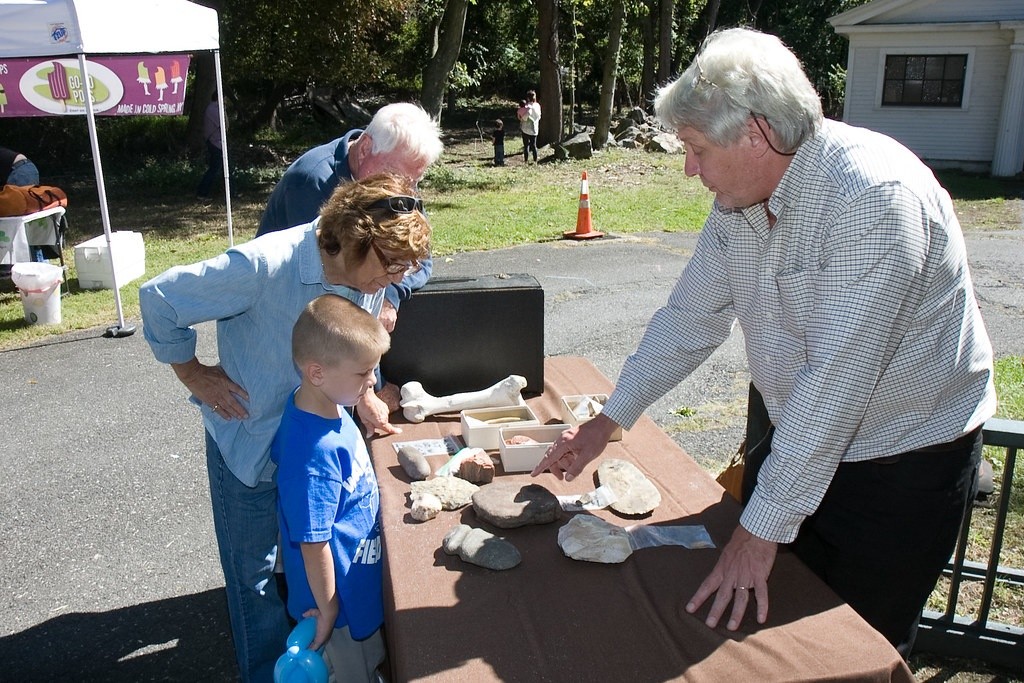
[562,171,603,240]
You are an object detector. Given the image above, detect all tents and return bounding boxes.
[0,1,234,339]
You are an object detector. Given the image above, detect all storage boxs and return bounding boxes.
[75,231,145,290]
[499,424,572,472]
[461,405,540,450]
[561,394,622,441]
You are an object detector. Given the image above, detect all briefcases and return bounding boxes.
[379,272,544,395]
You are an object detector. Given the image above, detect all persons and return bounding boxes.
[491,118,505,167]
[517,90,541,166]
[529,28,998,657]
[272,293,392,682]
[255,102,444,236]
[139,172,431,683]
[0,147,44,262]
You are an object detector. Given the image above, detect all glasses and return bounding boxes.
[689,54,749,111]
[370,240,422,277]
[367,195,427,219]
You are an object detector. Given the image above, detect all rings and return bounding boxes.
[212,405,220,411]
[736,586,746,591]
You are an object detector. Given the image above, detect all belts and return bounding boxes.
[11,160,29,172]
[869,424,984,464]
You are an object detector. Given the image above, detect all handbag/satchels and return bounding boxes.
[0,184,68,217]
[715,439,747,502]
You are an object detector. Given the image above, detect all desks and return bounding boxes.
[0,206,71,298]
[359,355,918,683]
